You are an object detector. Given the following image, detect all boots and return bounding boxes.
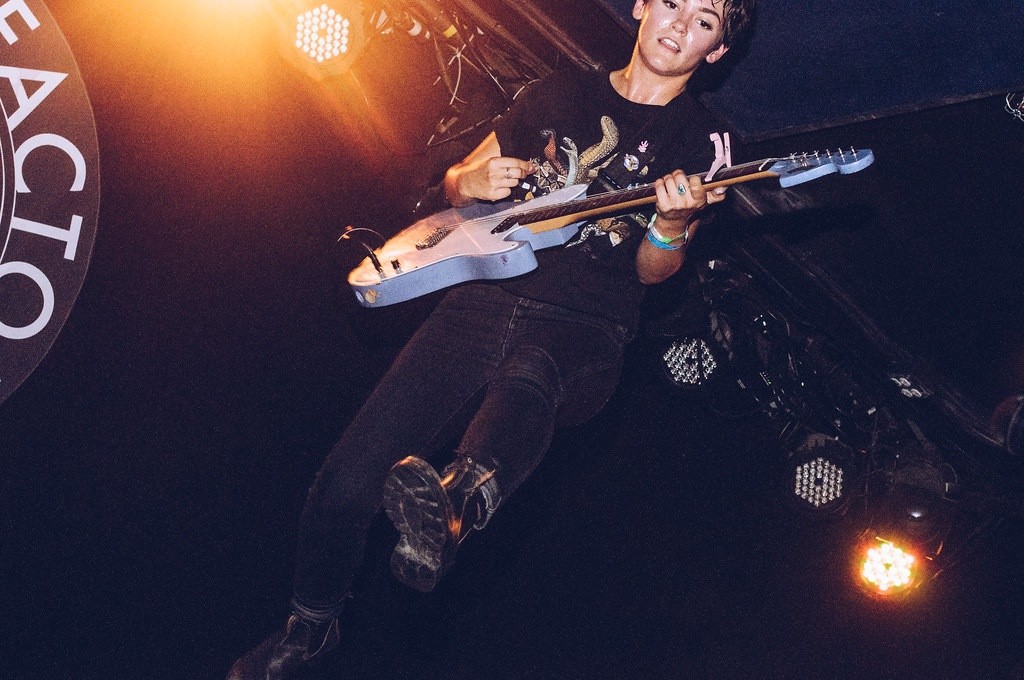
[383,454,490,592]
[226,613,339,680]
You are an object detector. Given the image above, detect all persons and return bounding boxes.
[228,0,762,680]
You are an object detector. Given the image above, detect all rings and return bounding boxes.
[504,165,510,181]
[676,182,690,196]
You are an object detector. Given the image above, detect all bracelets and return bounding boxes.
[646,220,690,251]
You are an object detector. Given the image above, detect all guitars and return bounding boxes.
[348,145,876,309]
